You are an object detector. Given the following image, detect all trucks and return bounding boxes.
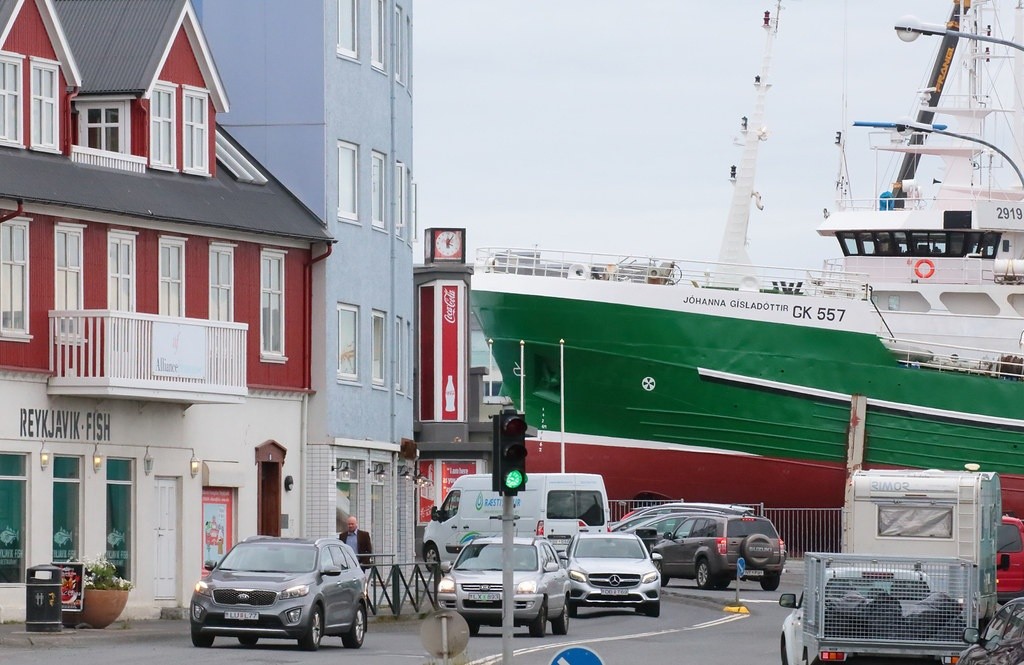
[778,552,980,665]
[842,463,1010,629]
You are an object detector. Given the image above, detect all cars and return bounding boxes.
[609,501,756,551]
[955,597,1024,665]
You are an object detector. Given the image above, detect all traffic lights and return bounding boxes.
[498,408,528,497]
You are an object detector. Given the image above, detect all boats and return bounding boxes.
[474,0,1023,550]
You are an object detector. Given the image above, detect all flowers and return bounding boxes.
[82,552,133,591]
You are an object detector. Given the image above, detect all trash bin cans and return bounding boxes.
[25,564,64,633]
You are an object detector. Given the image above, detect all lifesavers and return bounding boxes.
[913,258,935,279]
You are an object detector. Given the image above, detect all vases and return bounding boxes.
[62,589,129,629]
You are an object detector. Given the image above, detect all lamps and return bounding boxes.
[93,442,102,474]
[392,465,415,480]
[190,449,201,478]
[414,469,427,482]
[144,446,153,475]
[40,439,51,471]
[332,461,354,475]
[368,463,390,480]
[424,477,432,484]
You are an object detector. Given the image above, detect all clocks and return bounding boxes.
[431,227,466,263]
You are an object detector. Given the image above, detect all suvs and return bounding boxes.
[436,536,573,639]
[650,514,789,591]
[190,535,368,651]
[560,532,664,618]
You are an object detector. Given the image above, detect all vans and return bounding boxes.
[423,472,610,573]
[995,515,1024,605]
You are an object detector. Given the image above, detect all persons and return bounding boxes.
[339,516,372,574]
